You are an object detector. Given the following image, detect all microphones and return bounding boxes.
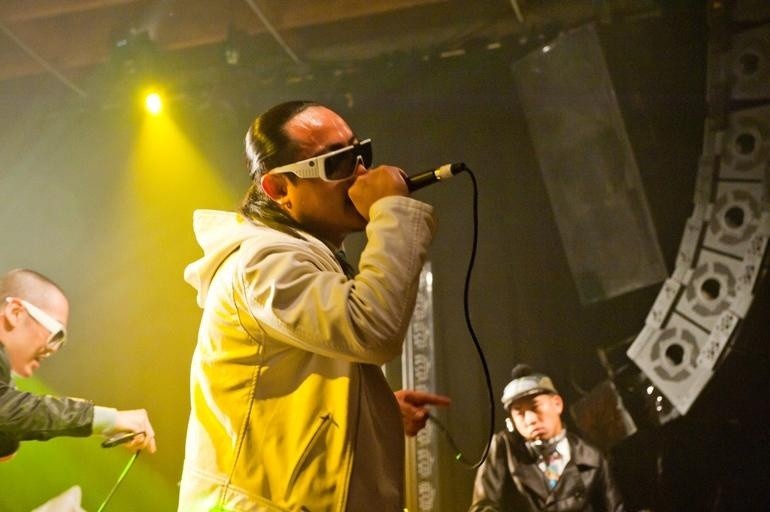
[405,161,465,193]
[101,432,133,448]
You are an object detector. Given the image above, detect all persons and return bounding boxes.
[176,98,453,511]
[467,364,625,511]
[1,265,158,512]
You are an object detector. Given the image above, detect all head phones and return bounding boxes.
[506,419,541,463]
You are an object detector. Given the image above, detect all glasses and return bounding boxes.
[267,137,375,185]
[6,293,67,352]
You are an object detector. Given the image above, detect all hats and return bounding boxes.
[501,370,557,411]
